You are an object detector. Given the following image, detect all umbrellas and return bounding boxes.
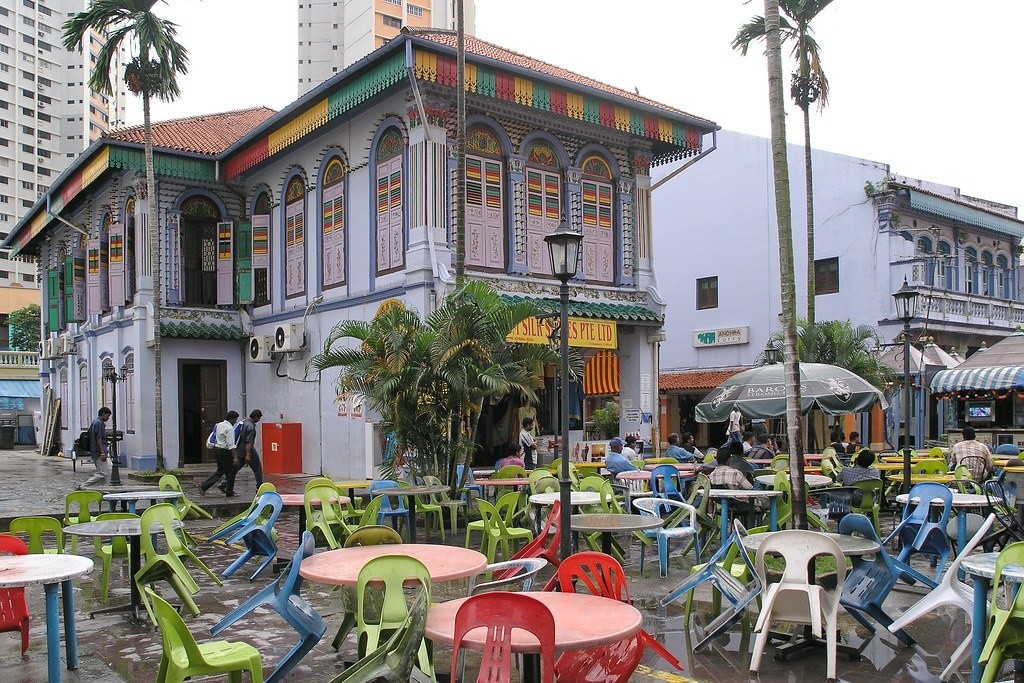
[695,359,888,424]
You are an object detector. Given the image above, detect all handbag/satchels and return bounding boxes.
[78,432,90,451]
[206,423,218,450]
[233,418,246,449]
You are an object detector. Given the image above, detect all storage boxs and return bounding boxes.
[261,422,303,475]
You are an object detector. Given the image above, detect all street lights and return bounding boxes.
[543,204,586,594]
[103,363,129,486]
[763,337,779,437]
[892,273,922,557]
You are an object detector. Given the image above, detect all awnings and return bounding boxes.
[930,365,1024,395]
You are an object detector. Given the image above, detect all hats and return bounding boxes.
[609,437,627,447]
[624,436,637,443]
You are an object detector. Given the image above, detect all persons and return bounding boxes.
[838,450,881,507]
[666,432,704,461]
[197,410,240,497]
[830,430,864,453]
[709,447,771,513]
[218,410,264,489]
[500,444,525,478]
[605,437,637,473]
[952,426,994,482]
[75,407,110,491]
[519,417,538,470]
[730,407,744,441]
[741,431,781,458]
[621,436,637,461]
[699,441,754,479]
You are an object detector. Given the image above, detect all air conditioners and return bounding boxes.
[38,192,42,197]
[47,338,63,358]
[40,33,44,37]
[249,335,277,363]
[39,158,44,163]
[59,337,77,355]
[39,341,51,360]
[39,83,45,90]
[273,322,305,351]
[39,101,45,108]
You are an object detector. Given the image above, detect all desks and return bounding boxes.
[476,477,530,528]
[472,469,534,504]
[423,591,644,683]
[61,517,185,626]
[958,551,1024,683]
[298,544,489,588]
[755,474,833,530]
[528,491,612,553]
[371,485,451,544]
[894,492,1002,582]
[255,493,351,575]
[742,529,881,664]
[748,459,772,463]
[645,463,704,492]
[772,465,823,471]
[886,474,968,483]
[992,453,1020,459]
[616,470,694,493]
[697,488,784,561]
[644,458,666,463]
[338,480,371,526]
[102,491,183,550]
[881,455,945,464]
[550,513,666,598]
[804,456,823,466]
[995,459,1009,466]
[576,462,606,475]
[873,462,916,509]
[0,554,95,683]
[1002,465,1024,473]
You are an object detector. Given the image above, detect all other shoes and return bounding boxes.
[73,484,83,491]
[226,493,241,498]
[217,482,227,493]
[197,482,205,497]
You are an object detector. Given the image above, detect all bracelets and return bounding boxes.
[101,453,105,455]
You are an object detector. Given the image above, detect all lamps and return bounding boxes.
[933,224,940,235]
[993,241,1002,250]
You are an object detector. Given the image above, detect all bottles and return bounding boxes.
[625,431,641,441]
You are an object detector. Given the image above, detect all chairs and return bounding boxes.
[79,432,90,450]
[0,444,1024,683]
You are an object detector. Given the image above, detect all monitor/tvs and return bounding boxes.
[965,401,995,422]
